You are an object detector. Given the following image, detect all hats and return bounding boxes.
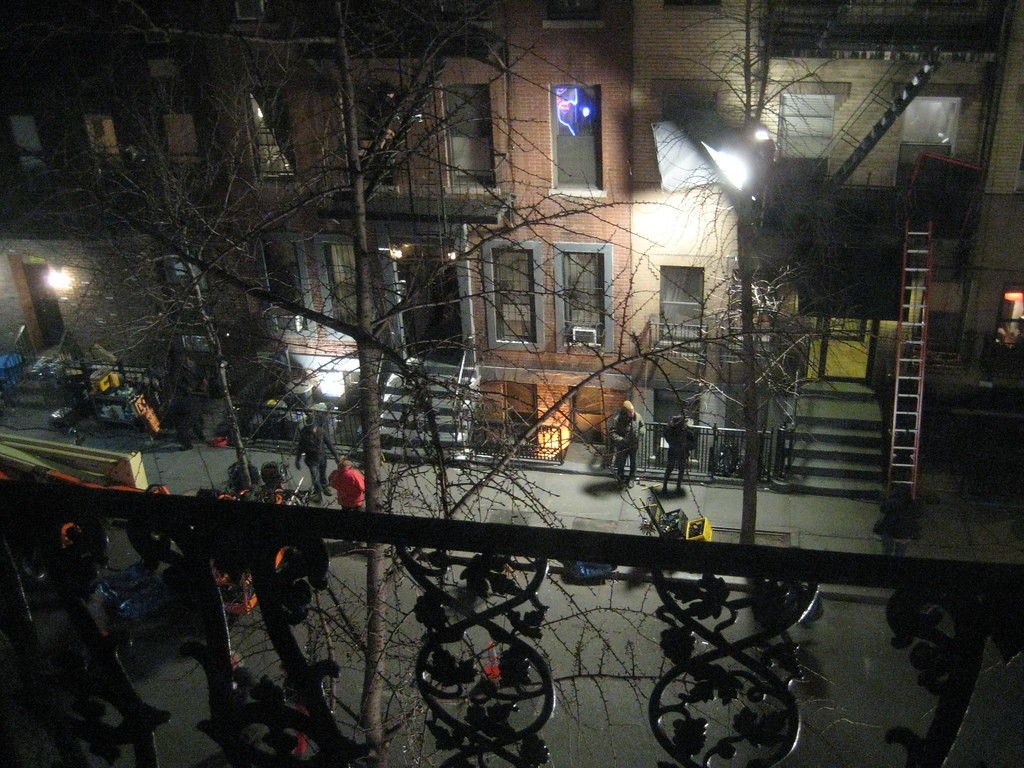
[622,400,634,412]
[303,415,318,428]
[672,416,682,423]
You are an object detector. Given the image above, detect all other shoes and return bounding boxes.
[628,481,634,487]
[323,488,332,496]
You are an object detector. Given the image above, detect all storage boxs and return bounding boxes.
[660,510,712,542]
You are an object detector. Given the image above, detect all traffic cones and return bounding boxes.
[484,640,501,679]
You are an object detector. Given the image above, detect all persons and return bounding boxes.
[162,372,205,450]
[663,417,696,490]
[874,487,921,557]
[609,400,646,488]
[295,418,340,496]
[329,459,366,511]
[996,322,1024,351]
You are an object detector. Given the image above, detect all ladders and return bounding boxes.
[884,217,934,502]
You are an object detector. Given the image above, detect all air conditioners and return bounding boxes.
[573,328,596,343]
